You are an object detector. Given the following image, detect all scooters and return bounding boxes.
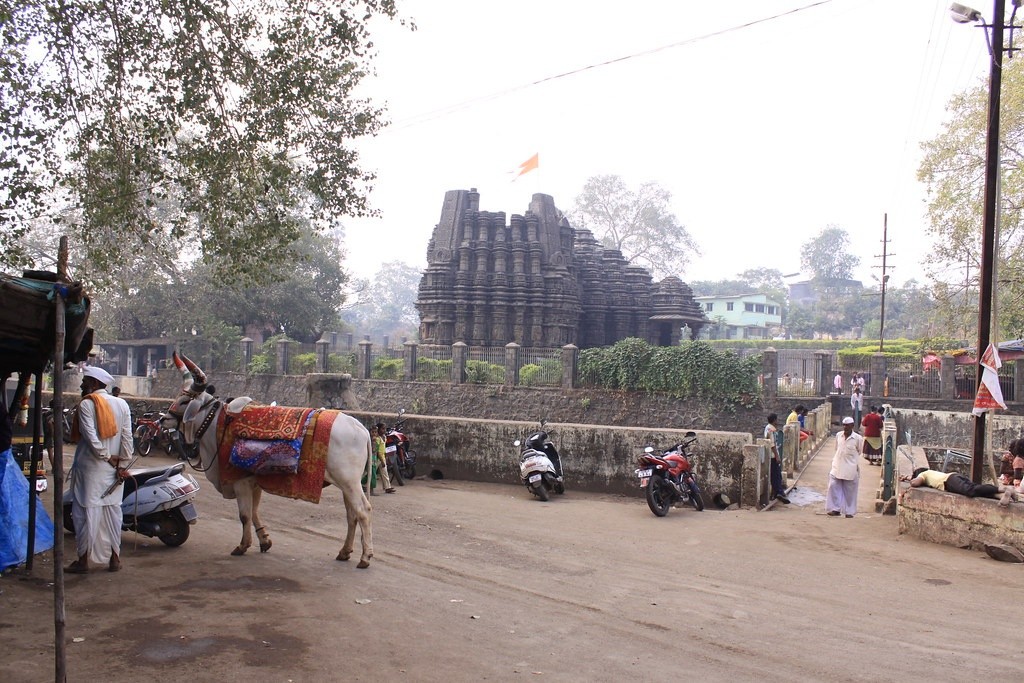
[63,462,200,547]
[513,417,565,502]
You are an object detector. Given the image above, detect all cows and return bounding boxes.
[159,350,374,569]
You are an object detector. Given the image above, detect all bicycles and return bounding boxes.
[61,405,78,443]
[129,399,200,461]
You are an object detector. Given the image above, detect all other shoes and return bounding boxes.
[61,560,89,574]
[845,514,854,518]
[776,493,791,504]
[827,511,841,516]
[108,562,123,572]
[385,489,396,493]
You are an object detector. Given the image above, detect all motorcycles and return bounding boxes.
[384,408,417,486]
[634,431,704,517]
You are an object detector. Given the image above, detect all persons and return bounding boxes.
[362,422,396,497]
[112,387,121,397]
[798,408,814,436]
[851,386,863,429]
[43,398,54,466]
[786,405,808,450]
[1000,438,1024,494]
[850,373,866,395]
[862,405,883,466]
[764,413,791,504]
[899,468,1018,507]
[783,372,798,387]
[826,416,864,519]
[876,406,885,421]
[63,364,133,572]
[834,371,842,394]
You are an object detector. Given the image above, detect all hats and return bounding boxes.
[842,416,854,424]
[81,365,116,386]
